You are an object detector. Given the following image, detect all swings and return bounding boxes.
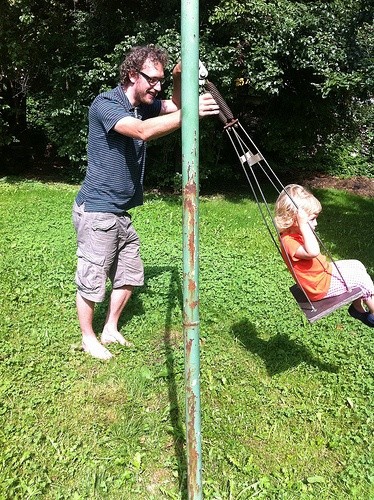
[204,78,367,323]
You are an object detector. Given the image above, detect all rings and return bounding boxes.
[209,104,213,110]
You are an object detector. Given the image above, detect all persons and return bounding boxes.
[71,44,220,361]
[272,184,373,329]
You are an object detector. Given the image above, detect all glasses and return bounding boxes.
[132,66,165,85]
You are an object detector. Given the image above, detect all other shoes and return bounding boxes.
[348,304,373,327]
[366,311,374,323]
